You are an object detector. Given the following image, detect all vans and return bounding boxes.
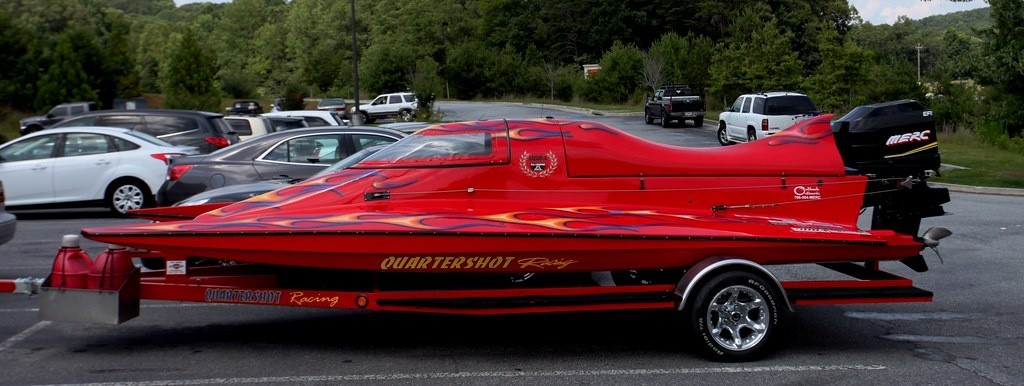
[224,115,275,144]
[49,111,241,158]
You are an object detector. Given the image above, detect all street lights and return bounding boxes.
[442,20,449,99]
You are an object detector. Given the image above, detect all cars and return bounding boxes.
[270,99,289,112]
[0,128,200,217]
[376,122,432,141]
[153,126,408,206]
[317,98,346,111]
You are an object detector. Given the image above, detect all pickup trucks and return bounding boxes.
[226,101,263,115]
[644,84,706,128]
[19,103,104,135]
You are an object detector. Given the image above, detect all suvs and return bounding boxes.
[350,93,419,121]
[259,112,346,158]
[717,92,820,146]
